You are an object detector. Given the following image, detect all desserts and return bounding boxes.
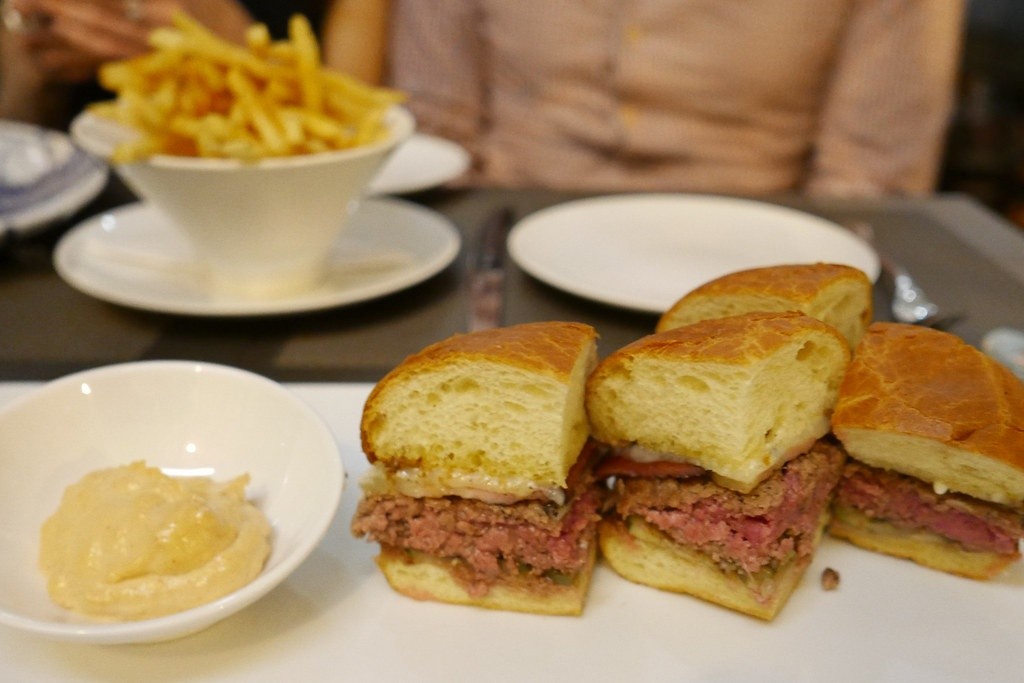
[42,460,270,619]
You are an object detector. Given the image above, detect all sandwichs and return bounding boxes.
[586,311,851,621]
[656,264,872,350]
[828,322,1024,582]
[353,324,601,613]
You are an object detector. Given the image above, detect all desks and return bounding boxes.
[0,190,1024,384]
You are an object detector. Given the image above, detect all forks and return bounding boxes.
[849,216,971,327]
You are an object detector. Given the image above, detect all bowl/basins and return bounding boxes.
[0,358,348,646]
[77,95,418,285]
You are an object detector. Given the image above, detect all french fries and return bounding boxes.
[88,9,406,166]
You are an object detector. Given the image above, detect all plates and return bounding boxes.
[51,195,465,319]
[366,132,471,193]
[505,191,884,314]
[0,120,109,234]
[0,380,1024,683]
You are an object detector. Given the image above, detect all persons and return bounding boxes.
[383,0,965,200]
[1,0,392,141]
